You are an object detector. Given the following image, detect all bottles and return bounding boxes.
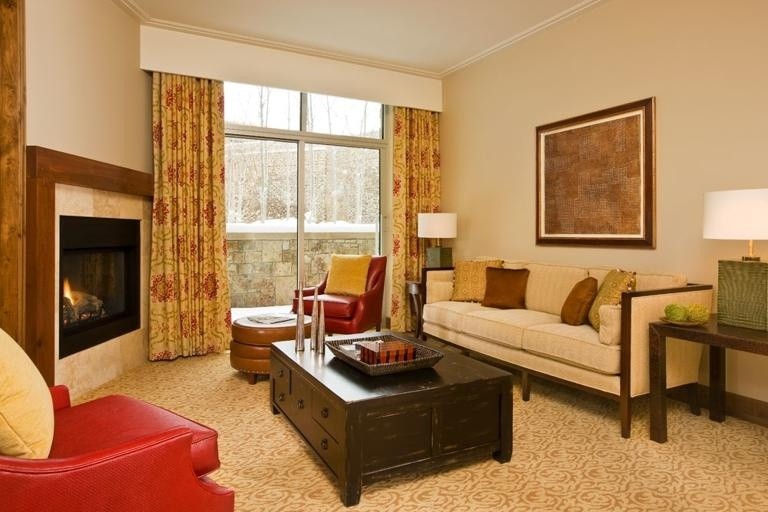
[316,297,326,354]
[310,287,319,350]
[295,280,304,351]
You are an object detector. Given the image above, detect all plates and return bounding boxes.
[660,317,703,326]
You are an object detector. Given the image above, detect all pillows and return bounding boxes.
[0,327,59,462]
[480,266,530,310]
[587,267,637,333]
[560,276,599,327]
[448,259,505,305]
[323,253,373,297]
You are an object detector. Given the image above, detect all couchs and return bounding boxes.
[420,261,715,440]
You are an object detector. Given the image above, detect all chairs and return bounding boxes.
[0,382,237,512]
[291,254,388,336]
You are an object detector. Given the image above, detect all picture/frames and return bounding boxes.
[533,95,658,252]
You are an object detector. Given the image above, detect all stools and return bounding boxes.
[227,314,313,386]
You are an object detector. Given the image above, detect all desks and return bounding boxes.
[270,328,516,509]
[406,281,426,339]
[647,312,767,445]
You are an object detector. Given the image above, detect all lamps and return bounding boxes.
[702,187,767,333]
[416,212,458,268]
